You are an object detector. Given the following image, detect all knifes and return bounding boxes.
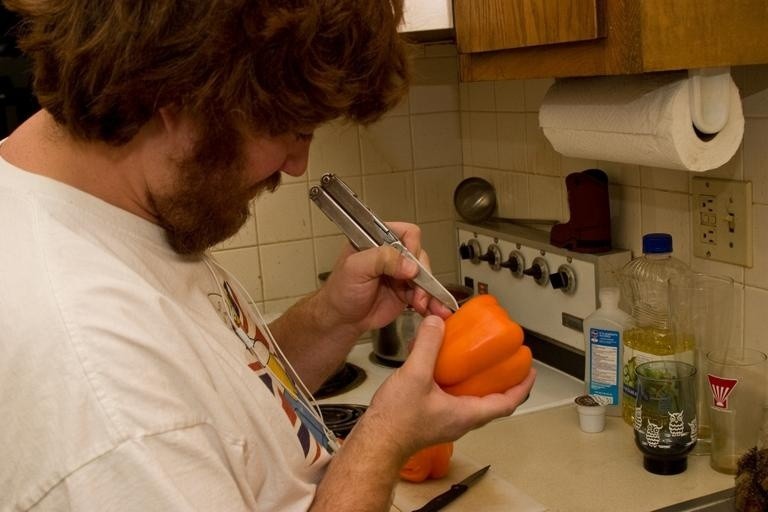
[310,172,460,317]
[406,464,493,511]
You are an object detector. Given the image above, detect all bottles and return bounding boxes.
[582,232,698,433]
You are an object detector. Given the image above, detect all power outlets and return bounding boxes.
[689,175,753,268]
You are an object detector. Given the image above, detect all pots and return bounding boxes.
[369,283,474,362]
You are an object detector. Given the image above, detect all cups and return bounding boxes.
[575,394,612,433]
[634,272,768,476]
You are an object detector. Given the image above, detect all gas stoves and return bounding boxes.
[312,325,633,440]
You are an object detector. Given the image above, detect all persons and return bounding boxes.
[0,1,538,512]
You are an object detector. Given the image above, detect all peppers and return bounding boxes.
[409,290,534,397]
[400,441,454,483]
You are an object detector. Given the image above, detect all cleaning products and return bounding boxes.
[583,286,634,406]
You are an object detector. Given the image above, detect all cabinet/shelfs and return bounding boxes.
[453,1,767,82]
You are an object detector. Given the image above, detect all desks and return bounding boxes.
[389,390,736,512]
